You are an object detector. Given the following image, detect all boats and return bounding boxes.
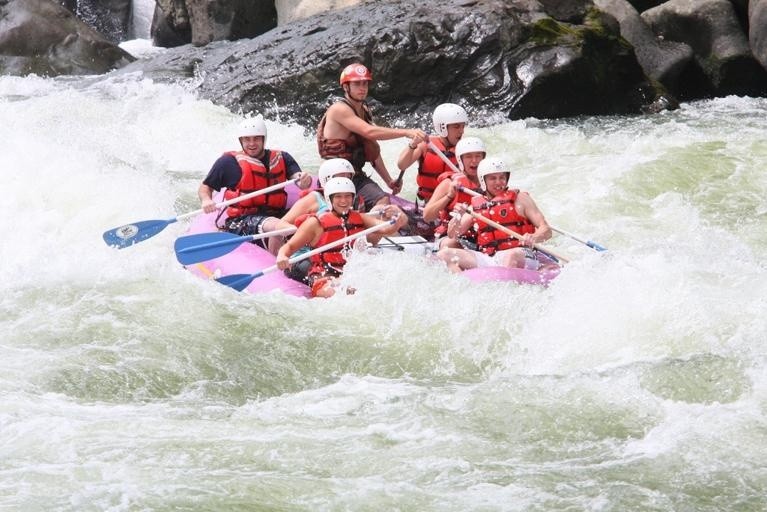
[181,188,560,300]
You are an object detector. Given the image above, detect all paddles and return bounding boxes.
[174,208,386,265]
[216,219,396,293]
[456,185,608,253]
[103,180,297,248]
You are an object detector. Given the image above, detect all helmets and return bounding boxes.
[452,138,486,171]
[339,63,372,84]
[476,157,510,192]
[319,158,356,211]
[431,103,468,138]
[237,118,267,144]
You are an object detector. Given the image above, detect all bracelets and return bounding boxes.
[446,195,453,199]
[408,143,418,150]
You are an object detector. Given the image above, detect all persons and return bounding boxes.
[276,176,407,298]
[198,117,313,257]
[268,158,396,254]
[318,63,419,211]
[423,136,488,247]
[436,157,553,272]
[397,103,470,202]
